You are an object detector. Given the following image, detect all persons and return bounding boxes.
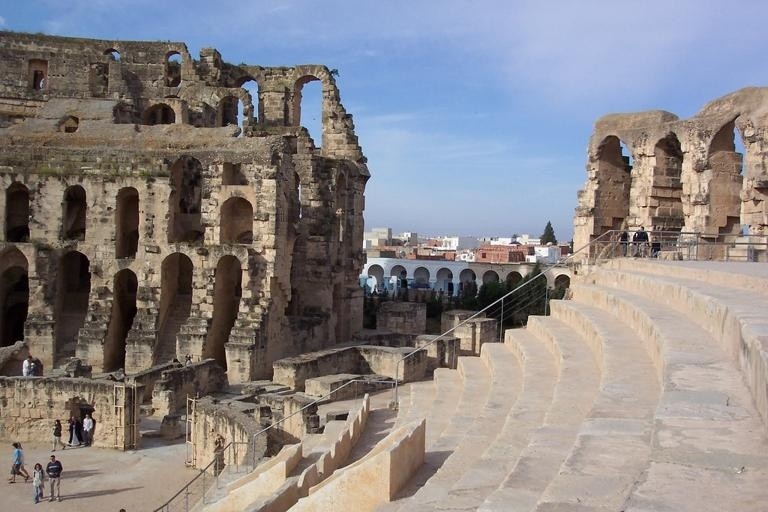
[22,355,36,376]
[8,413,93,504]
[620,226,661,259]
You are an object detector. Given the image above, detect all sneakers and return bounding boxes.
[55,497,61,502]
[61,445,66,450]
[32,501,38,505]
[48,497,54,502]
[8,477,17,484]
[24,475,30,484]
[51,449,57,452]
